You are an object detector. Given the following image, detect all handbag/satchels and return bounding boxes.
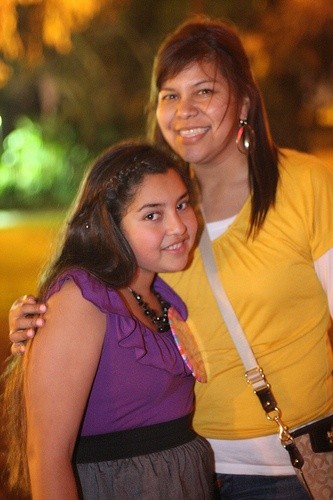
[285,415,333,500]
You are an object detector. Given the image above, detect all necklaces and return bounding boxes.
[124,284,170,334]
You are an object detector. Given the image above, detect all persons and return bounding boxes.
[9,17,333,500]
[20,140,223,500]
